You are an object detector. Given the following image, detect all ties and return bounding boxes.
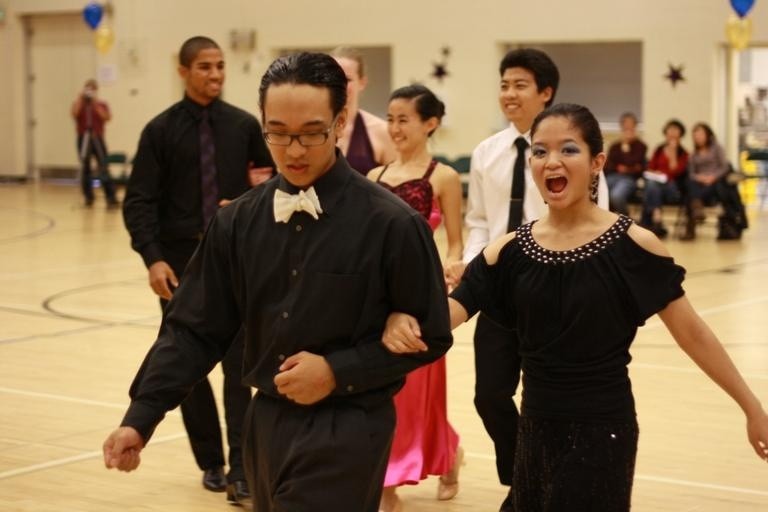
[199,110,220,237]
[508,138,529,233]
[272,187,322,224]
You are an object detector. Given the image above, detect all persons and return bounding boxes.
[119,34,282,501]
[380,100,768,511]
[361,78,467,511]
[599,85,767,243]
[69,78,122,210]
[325,55,409,175]
[101,48,453,512]
[463,48,609,487]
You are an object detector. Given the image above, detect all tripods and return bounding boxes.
[71,107,117,207]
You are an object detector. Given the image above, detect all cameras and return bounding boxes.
[83,90,95,103]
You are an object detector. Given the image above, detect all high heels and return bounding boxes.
[436,446,466,501]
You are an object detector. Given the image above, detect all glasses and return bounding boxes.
[262,115,341,147]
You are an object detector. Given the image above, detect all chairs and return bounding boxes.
[432,152,686,240]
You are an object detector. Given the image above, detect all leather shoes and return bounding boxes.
[202,463,227,492]
[224,470,251,501]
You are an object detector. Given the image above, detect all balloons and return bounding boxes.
[727,0,756,18]
[724,13,753,53]
[90,25,116,54]
[81,2,102,30]
